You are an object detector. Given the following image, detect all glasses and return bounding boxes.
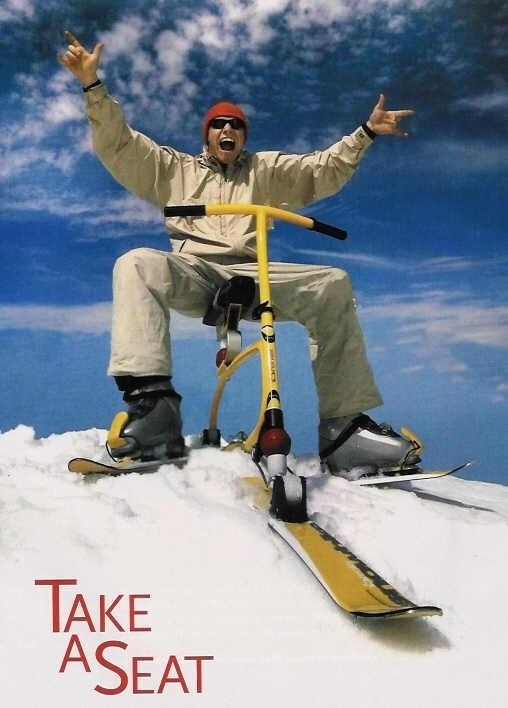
[211,117,243,128]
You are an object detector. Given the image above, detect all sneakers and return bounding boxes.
[109,398,181,456]
[321,415,421,469]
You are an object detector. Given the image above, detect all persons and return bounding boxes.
[54,29,423,478]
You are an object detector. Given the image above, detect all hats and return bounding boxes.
[203,102,248,146]
